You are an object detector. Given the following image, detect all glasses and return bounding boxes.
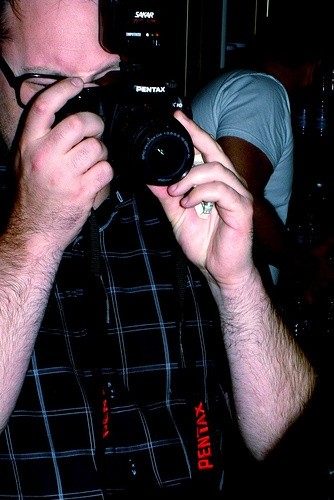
[0,56,69,111]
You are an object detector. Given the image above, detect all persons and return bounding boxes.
[187,6,333,364]
[0,0,334,500]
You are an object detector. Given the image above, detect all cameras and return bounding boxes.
[57,61,195,186]
